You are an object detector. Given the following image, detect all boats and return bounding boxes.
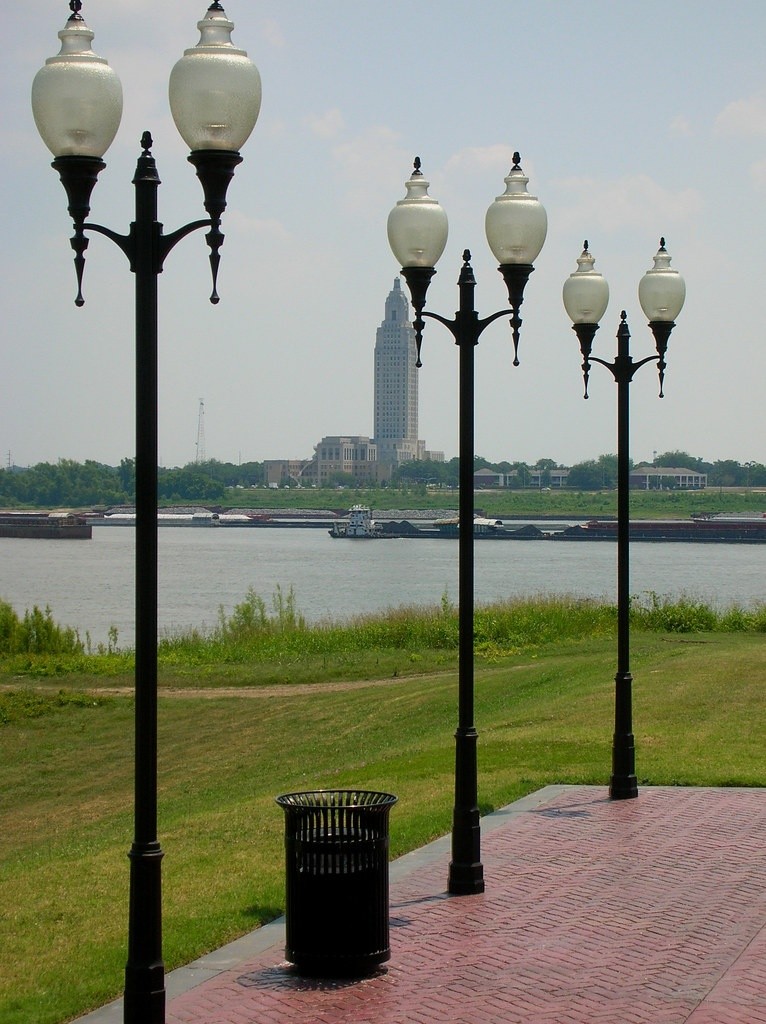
[327,504,389,539]
[0,512,93,540]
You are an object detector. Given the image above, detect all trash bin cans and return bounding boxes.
[273,788,399,975]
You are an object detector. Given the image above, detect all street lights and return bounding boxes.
[563,233,688,800]
[386,147,548,895]
[25,0,261,1024]
